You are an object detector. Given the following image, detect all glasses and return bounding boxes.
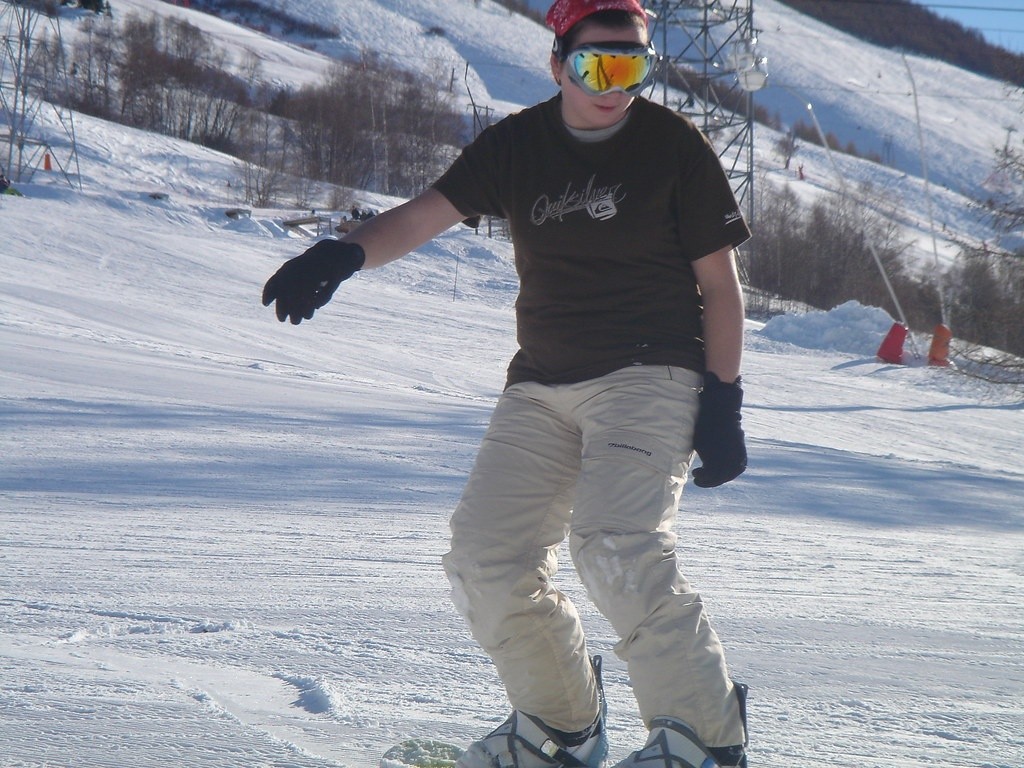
[565,40,658,98]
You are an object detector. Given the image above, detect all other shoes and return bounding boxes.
[607,683,750,768]
[449,655,607,768]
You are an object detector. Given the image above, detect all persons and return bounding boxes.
[262,0,751,768]
[340,206,374,223]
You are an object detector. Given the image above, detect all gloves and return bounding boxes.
[262,238,366,327]
[691,378,748,488]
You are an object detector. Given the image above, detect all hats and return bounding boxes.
[546,0,650,38]
